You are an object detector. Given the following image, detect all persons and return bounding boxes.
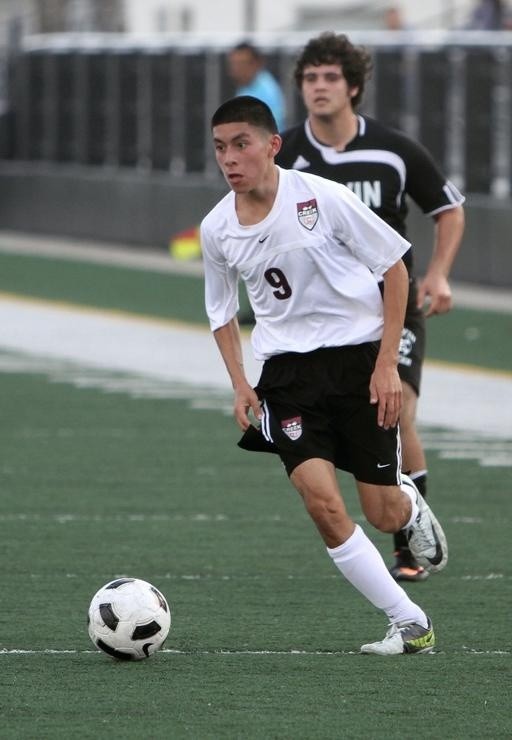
[198,96,448,657]
[229,40,291,136]
[271,33,466,584]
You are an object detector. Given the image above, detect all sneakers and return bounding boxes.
[396,473,449,574]
[359,615,437,657]
[389,545,431,582]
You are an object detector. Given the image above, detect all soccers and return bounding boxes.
[88,577,172,660]
